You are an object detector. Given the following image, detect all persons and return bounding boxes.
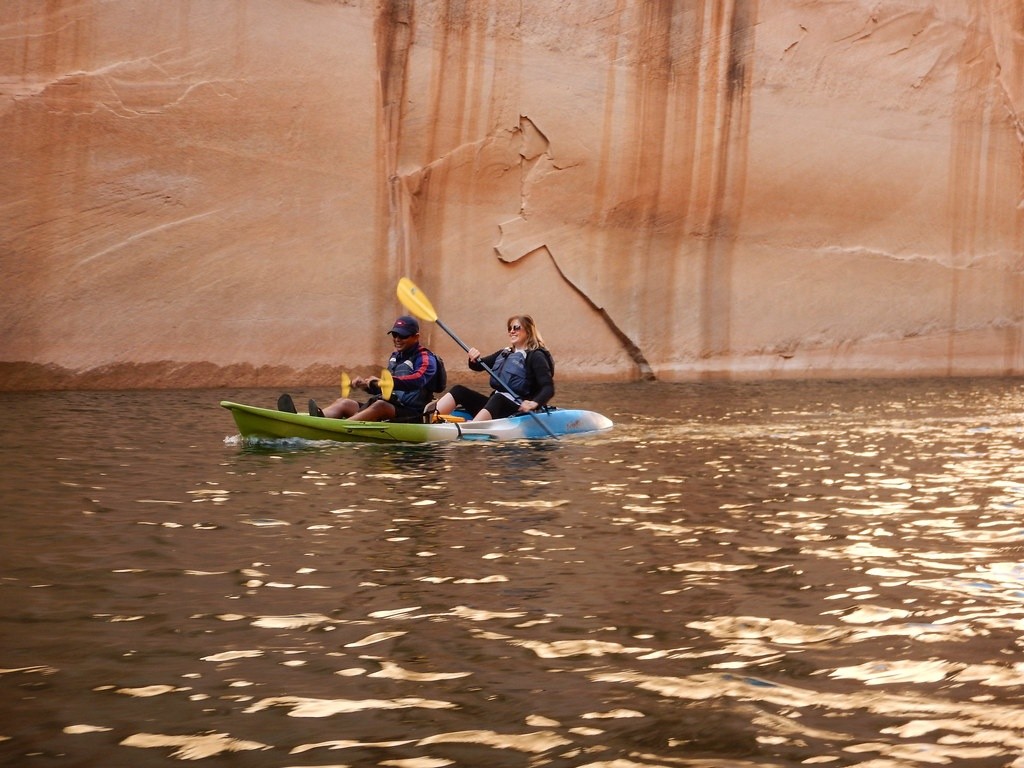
[277,316,436,422]
[423,314,554,424]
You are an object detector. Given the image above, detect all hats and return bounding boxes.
[387,315,419,336]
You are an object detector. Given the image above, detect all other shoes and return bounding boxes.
[277,393,297,413]
[309,399,325,417]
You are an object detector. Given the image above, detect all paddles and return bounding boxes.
[339,367,396,401]
[392,274,561,442]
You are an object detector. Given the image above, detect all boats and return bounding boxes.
[218,399,619,453]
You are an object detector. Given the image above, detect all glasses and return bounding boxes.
[392,332,415,339]
[508,325,522,331]
